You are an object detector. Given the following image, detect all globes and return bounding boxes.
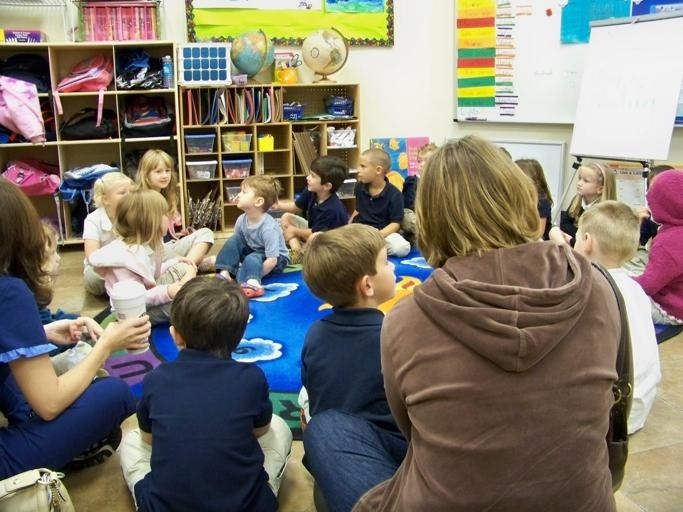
[231,29,275,84]
[302,27,348,85]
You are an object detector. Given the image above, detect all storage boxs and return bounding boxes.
[185,157,218,180]
[222,156,253,178]
[335,178,357,199]
[327,129,356,147]
[221,134,252,153]
[184,131,216,154]
[223,182,241,203]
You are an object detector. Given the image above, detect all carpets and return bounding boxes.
[50,247,683,441]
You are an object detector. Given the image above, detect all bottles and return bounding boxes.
[162,56,173,87]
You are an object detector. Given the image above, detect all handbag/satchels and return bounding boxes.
[0,467,74,512]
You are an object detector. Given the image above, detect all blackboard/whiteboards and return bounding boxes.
[569,16,683,161]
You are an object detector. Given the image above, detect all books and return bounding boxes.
[80,6,155,42]
[183,88,282,125]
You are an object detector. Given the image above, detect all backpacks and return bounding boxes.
[1,158,60,195]
[61,108,122,139]
[57,164,119,203]
[57,52,113,125]
[124,94,174,137]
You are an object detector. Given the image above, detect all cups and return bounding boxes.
[276,67,297,84]
[107,281,149,356]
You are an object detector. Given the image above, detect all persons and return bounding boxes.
[81,172,137,297]
[117,272,293,510]
[548,158,618,252]
[31,220,108,384]
[571,199,662,437]
[400,141,440,240]
[299,135,636,510]
[82,189,197,330]
[213,173,290,298]
[0,174,154,482]
[295,223,405,440]
[513,158,552,242]
[344,147,411,259]
[621,165,676,279]
[628,169,683,325]
[270,155,348,262]
[132,148,214,270]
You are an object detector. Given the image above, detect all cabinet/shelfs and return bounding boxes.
[179,83,361,240]
[0,40,186,246]
